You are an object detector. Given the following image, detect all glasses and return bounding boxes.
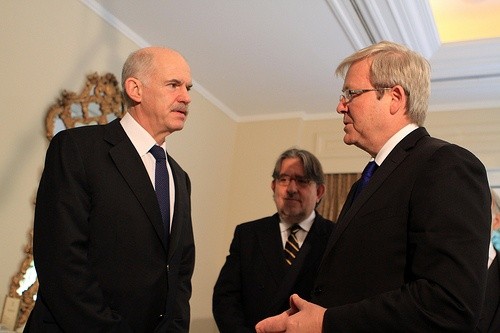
[339,88,392,101]
[274,174,315,189]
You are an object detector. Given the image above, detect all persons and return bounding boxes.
[477,241,500,333]
[212,149,336,333]
[23,46,195,333]
[255,41,492,333]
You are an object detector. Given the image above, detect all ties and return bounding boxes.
[150,144,171,239]
[284,223,300,267]
[349,159,378,210]
